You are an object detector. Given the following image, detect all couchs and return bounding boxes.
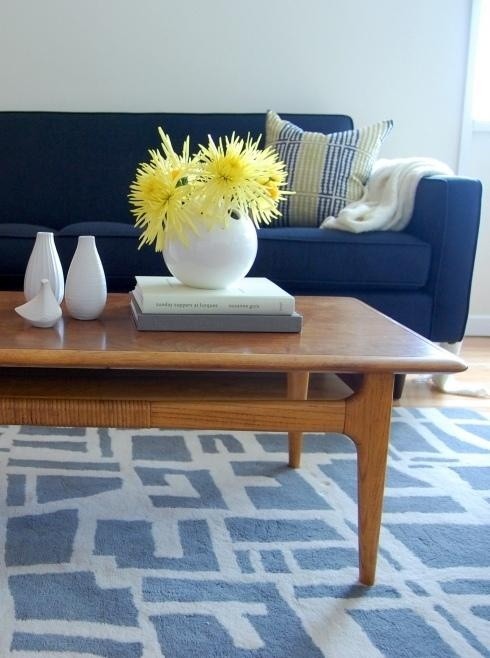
[1,111,483,400]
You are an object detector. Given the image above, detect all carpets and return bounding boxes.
[1,406,487,658]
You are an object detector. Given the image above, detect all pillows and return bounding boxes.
[263,111,394,227]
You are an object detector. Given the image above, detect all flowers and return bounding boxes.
[129,125,298,253]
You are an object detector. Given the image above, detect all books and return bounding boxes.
[131,275,296,317]
[126,290,304,333]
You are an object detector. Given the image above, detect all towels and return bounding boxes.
[318,156,456,234]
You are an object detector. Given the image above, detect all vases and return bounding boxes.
[22,230,109,322]
[163,197,258,290]
